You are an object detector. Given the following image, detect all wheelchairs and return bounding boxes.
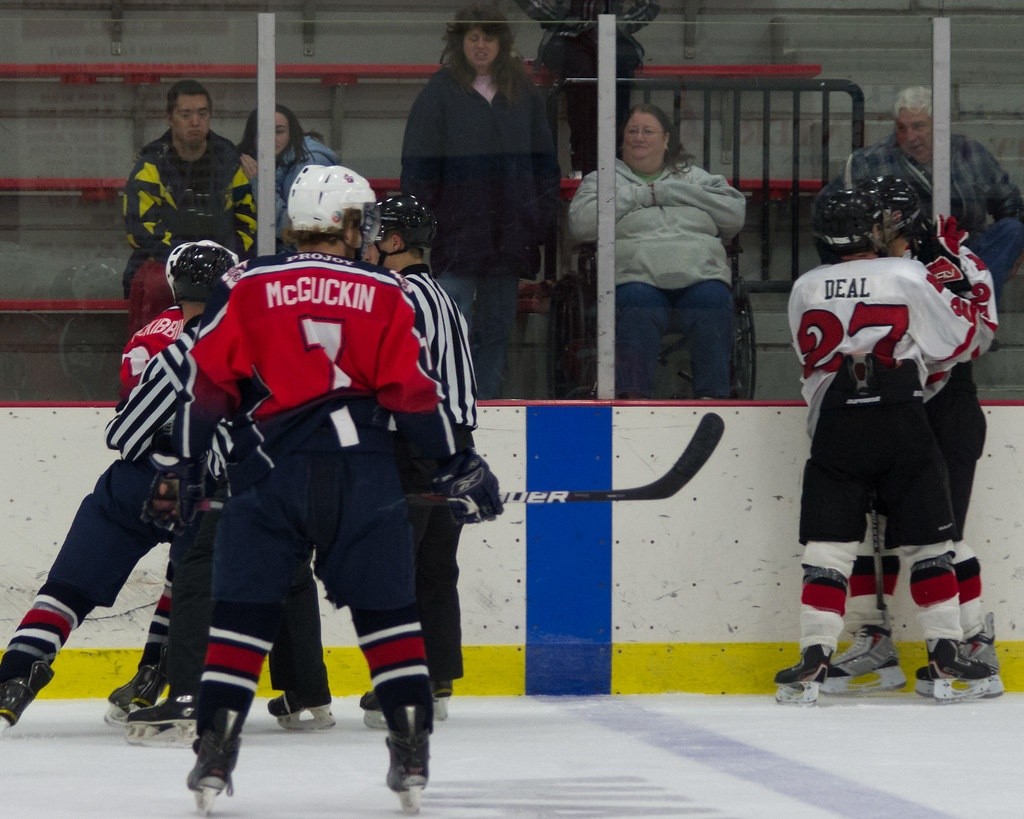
[546,232,759,400]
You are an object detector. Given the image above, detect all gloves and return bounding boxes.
[432,447,504,527]
[140,464,203,537]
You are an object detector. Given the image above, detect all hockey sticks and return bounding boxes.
[866,478,890,612]
[151,411,726,511]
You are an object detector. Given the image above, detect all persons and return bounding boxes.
[401,2,561,400]
[123,77,257,341]
[517,0,660,167]
[239,107,341,253]
[845,84,1024,304]
[567,105,745,398]
[2,165,501,818]
[772,177,1002,705]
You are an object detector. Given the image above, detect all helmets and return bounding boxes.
[165,239,238,303]
[859,175,926,239]
[375,194,436,248]
[286,164,376,234]
[809,189,874,260]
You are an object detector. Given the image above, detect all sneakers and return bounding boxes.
[387,707,427,812]
[363,677,453,729]
[770,643,834,705]
[187,710,244,812]
[105,666,168,726]
[833,623,904,691]
[1,661,56,737]
[912,635,969,695]
[931,615,1005,697]
[126,675,200,748]
[268,669,334,730]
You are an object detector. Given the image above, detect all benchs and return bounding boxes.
[0,61,828,406]
